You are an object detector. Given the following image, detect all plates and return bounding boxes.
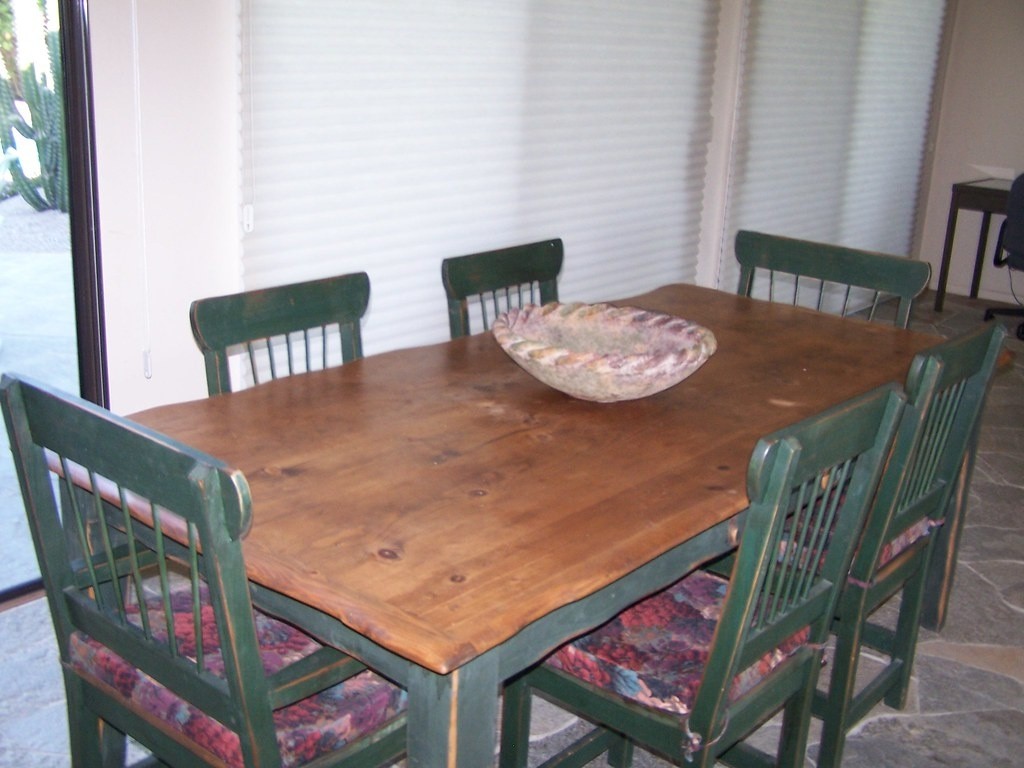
[491,302,717,404]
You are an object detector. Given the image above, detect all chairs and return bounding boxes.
[442,238,564,341]
[734,230,932,330]
[0,371,409,768]
[499,379,908,768]
[190,272,371,397]
[738,320,1011,768]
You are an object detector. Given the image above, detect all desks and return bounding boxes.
[43,283,1014,768]
[935,178,1015,314]
